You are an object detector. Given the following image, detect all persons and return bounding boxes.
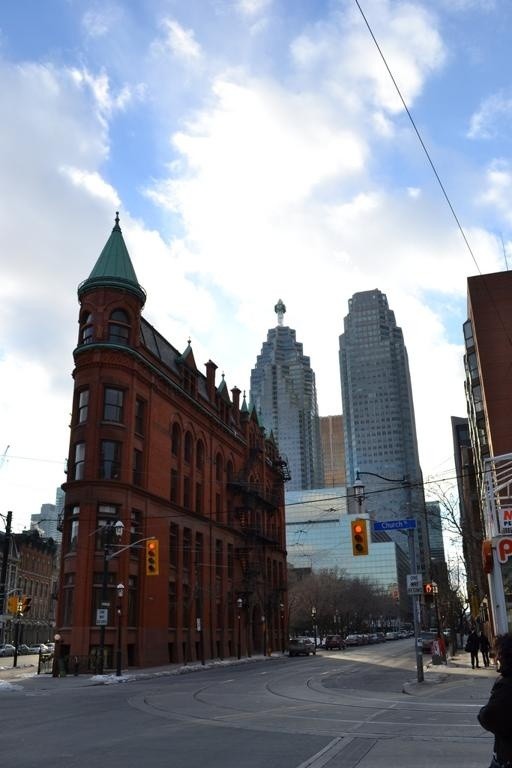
[464,627,512,768]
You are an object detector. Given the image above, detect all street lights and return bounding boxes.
[310,605,317,648]
[333,607,396,632]
[113,583,124,678]
[96,518,124,676]
[236,598,244,660]
[431,580,443,637]
[354,466,423,686]
[280,602,285,654]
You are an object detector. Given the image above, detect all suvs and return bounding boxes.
[326,635,344,650]
[288,637,316,656]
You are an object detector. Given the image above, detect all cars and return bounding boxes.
[1,643,17,658]
[344,628,414,647]
[416,624,451,654]
[14,640,55,656]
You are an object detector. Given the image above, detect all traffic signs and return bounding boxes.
[406,573,423,595]
[373,519,417,530]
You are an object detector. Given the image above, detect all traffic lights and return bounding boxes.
[21,595,32,612]
[423,583,432,595]
[351,519,367,555]
[145,539,159,575]
[7,596,16,613]
